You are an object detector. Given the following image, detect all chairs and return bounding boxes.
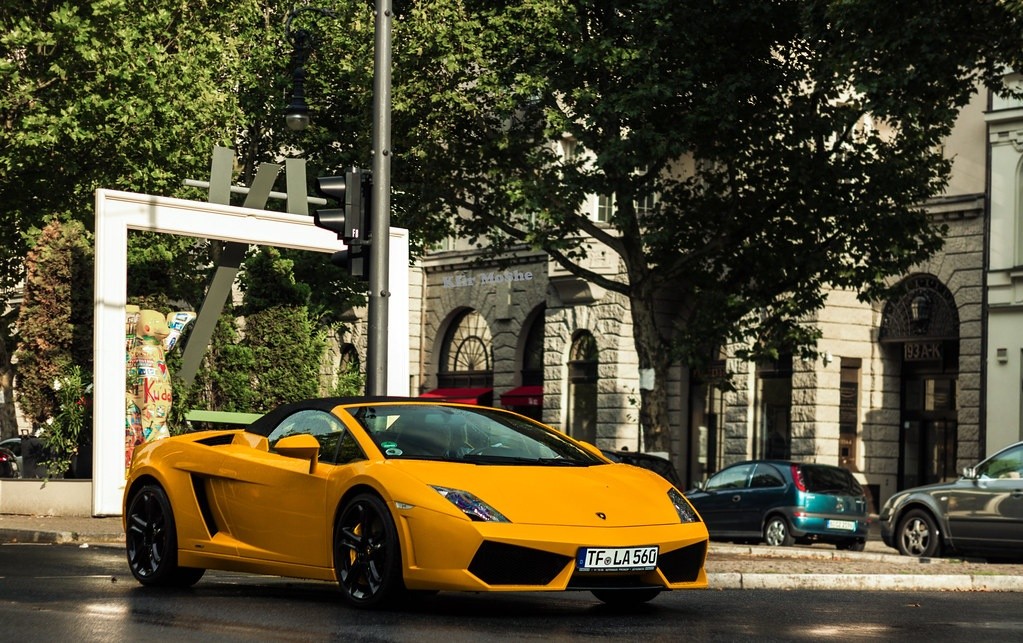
[394,419,452,458]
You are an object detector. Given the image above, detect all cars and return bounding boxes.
[680,458,870,551]
[0,436,31,479]
[879,440,1023,562]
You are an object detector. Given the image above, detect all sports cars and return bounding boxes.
[122,396,709,613]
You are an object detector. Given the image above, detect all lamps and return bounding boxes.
[909,288,930,334]
